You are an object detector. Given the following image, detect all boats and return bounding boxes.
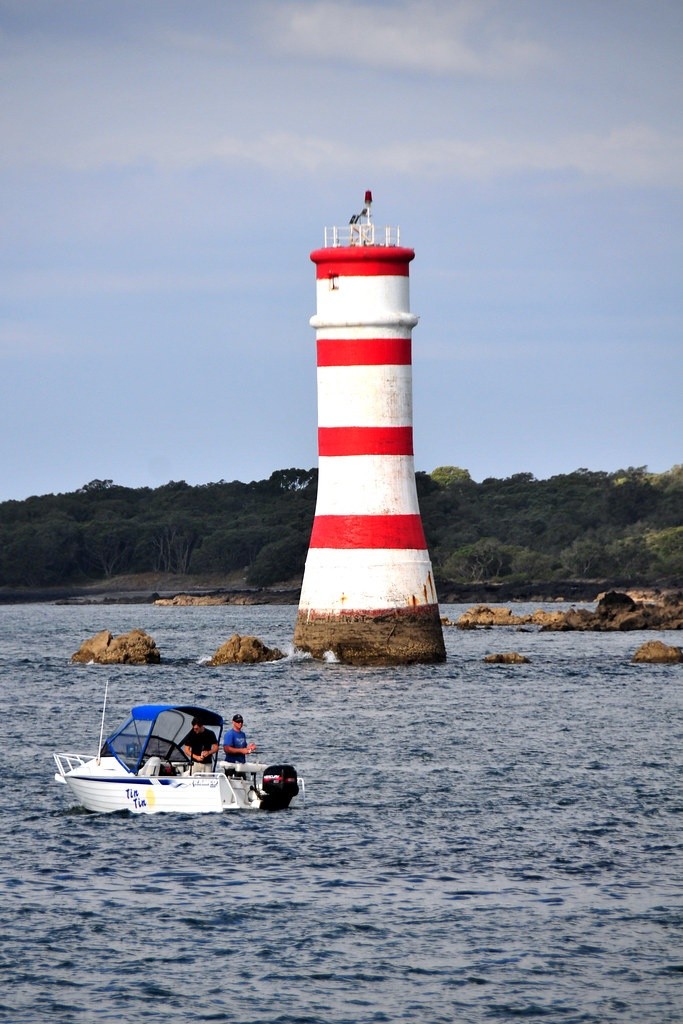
[51,680,305,816]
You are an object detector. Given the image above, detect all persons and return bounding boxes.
[184,718,219,776]
[223,714,256,780]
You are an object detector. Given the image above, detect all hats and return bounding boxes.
[233,714,243,723]
